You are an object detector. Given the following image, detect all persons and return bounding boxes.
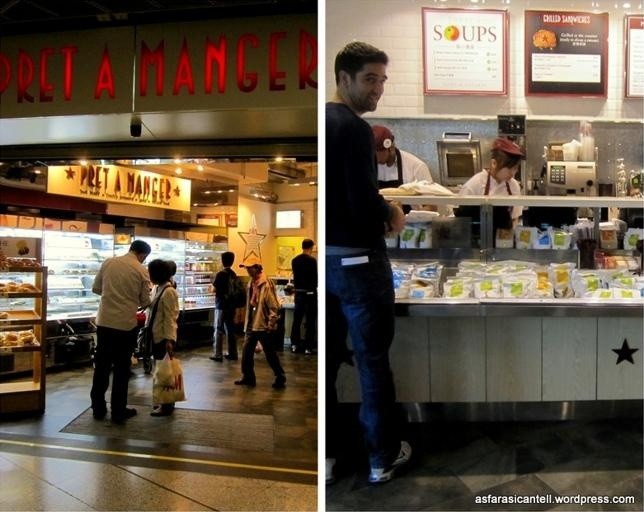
[233,258,286,389]
[209,251,240,362]
[371,123,432,232]
[290,239,318,355]
[453,136,522,250]
[90,240,151,424]
[325,40,412,483]
[145,259,179,417]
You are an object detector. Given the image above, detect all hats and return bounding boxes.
[372,126,395,152]
[492,137,525,160]
[239,256,261,267]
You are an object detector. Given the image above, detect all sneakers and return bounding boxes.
[367,441,412,483]
[326,458,336,485]
[92,344,318,422]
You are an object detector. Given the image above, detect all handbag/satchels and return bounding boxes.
[137,327,154,354]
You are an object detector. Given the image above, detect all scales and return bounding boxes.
[546,161,597,218]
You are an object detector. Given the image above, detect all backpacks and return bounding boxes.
[224,277,247,308]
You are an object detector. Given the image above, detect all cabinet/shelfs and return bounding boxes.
[0,263,48,421]
[43,223,227,322]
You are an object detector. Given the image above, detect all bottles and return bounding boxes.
[186,275,210,283]
[184,296,215,307]
[182,261,216,272]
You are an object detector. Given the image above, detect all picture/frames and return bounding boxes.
[524,9,609,99]
[421,6,510,97]
[623,14,644,101]
